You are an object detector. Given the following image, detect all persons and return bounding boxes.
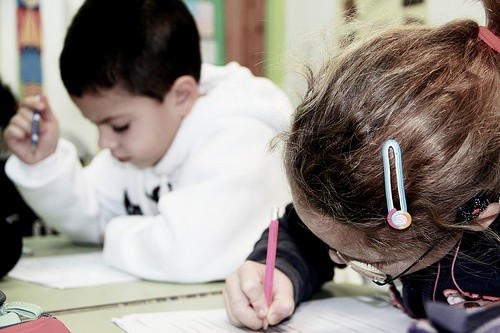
[223,0,500,333]
[3,0,295,284]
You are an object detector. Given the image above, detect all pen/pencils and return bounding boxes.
[262,205,280,333]
[30,93,41,156]
